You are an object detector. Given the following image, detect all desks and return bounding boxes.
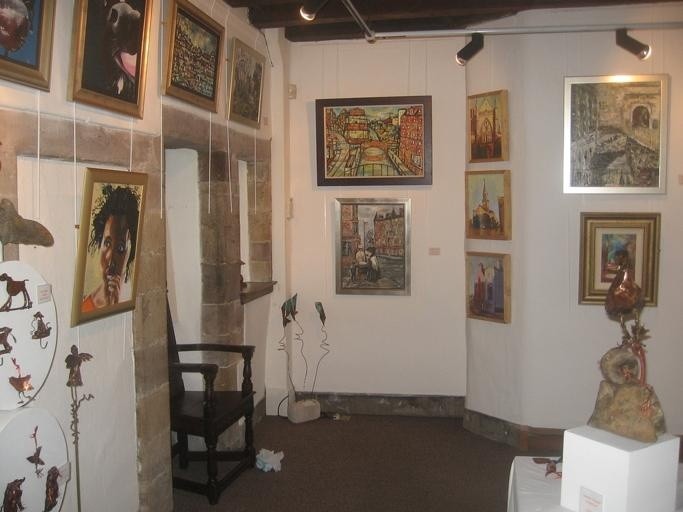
[510,456,683,510]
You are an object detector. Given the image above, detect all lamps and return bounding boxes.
[614,28,652,63]
[299,0,325,23]
[454,33,483,67]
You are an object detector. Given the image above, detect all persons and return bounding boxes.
[80,184,142,312]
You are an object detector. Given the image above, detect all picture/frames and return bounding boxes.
[560,70,670,310]
[69,167,148,329]
[462,87,514,326]
[0,2,267,130]
[313,95,433,297]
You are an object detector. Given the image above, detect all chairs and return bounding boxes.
[160,293,259,508]
